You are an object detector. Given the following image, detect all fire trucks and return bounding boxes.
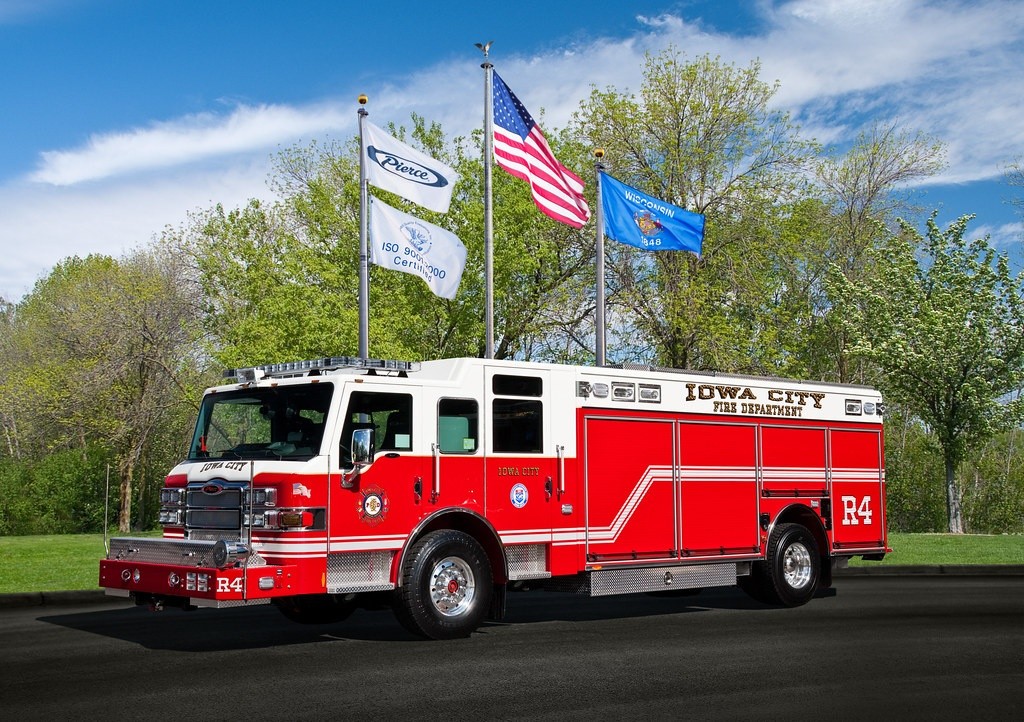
[95,355,894,639]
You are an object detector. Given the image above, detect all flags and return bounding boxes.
[596,170,706,260]
[485,67,591,231]
[359,193,469,301]
[359,117,459,214]
[293,483,311,498]
